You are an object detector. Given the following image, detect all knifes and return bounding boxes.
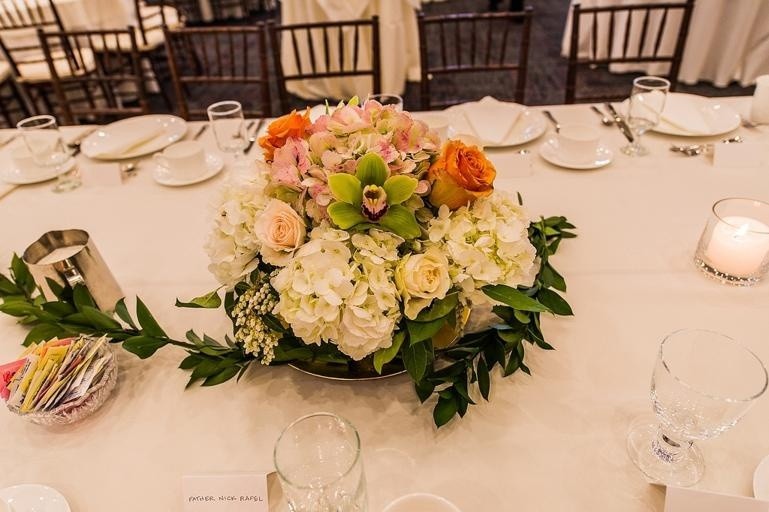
[603,99,634,144]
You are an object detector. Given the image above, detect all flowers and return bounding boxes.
[0,93,579,430]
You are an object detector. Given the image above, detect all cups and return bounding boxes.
[620,76,671,158]
[21,226,126,318]
[205,98,250,155]
[625,325,769,490]
[691,197,768,289]
[15,115,70,167]
[153,140,209,182]
[749,74,769,127]
[270,412,365,511]
[545,124,602,165]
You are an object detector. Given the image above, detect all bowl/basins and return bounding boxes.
[382,492,461,512]
[3,337,118,430]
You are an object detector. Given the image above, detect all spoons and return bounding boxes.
[668,136,746,158]
[590,105,613,126]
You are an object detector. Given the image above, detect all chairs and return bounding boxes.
[267,13,383,116]
[2,3,100,125]
[566,0,697,105]
[135,1,203,87]
[415,5,535,112]
[161,21,274,123]
[35,24,153,127]
[0,56,32,129]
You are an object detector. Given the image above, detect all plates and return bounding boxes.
[0,153,78,185]
[154,153,226,188]
[538,137,614,170]
[79,114,191,161]
[617,91,743,138]
[752,455,769,503]
[1,481,74,512]
[444,101,549,149]
